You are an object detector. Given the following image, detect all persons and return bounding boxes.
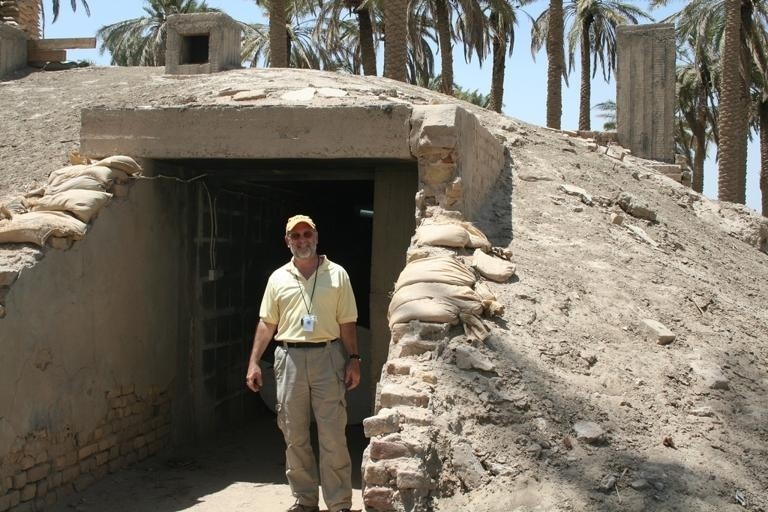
[245,214,361,512]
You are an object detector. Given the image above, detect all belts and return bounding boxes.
[276,340,341,349]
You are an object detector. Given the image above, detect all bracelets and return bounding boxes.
[348,354,360,362]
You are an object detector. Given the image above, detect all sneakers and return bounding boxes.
[287,505,320,512]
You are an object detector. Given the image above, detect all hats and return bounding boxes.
[285,214,316,231]
